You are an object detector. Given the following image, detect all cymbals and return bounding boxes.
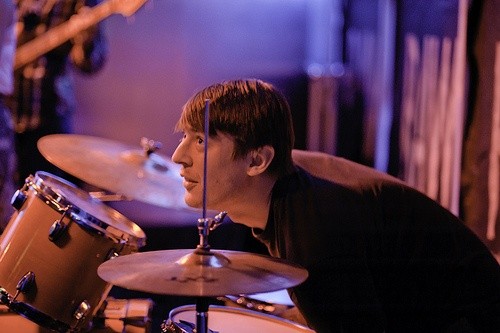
[97,249,308,296]
[37,133,221,214]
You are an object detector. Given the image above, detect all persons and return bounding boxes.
[0,0,106,196]
[172,79,500,333]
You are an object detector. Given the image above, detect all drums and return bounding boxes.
[73,298,153,332]
[162,305,316,333]
[217,289,312,330]
[0,172,146,333]
